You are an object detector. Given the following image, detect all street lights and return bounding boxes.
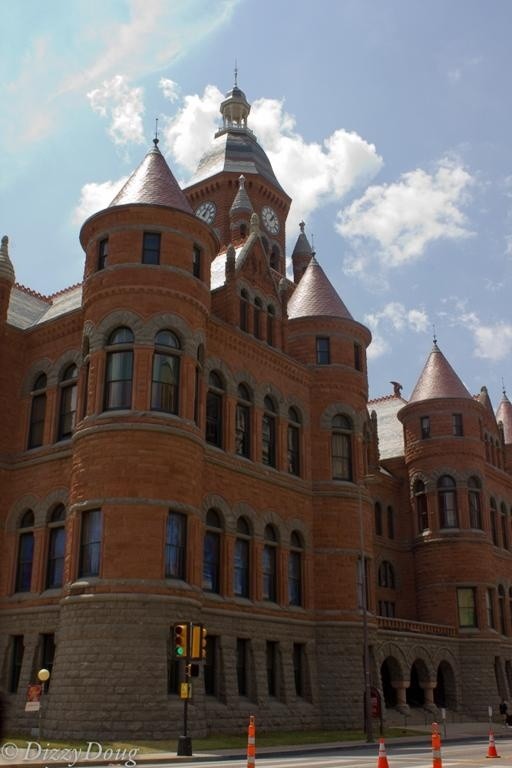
[356,474,377,742]
[37,666,51,747]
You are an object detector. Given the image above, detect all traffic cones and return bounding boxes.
[485,726,501,760]
[375,737,389,768]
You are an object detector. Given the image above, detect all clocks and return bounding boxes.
[261,204,282,235]
[194,201,217,226]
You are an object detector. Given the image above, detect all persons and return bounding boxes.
[499,698,507,726]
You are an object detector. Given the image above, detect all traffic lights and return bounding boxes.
[172,622,189,659]
[192,625,207,659]
